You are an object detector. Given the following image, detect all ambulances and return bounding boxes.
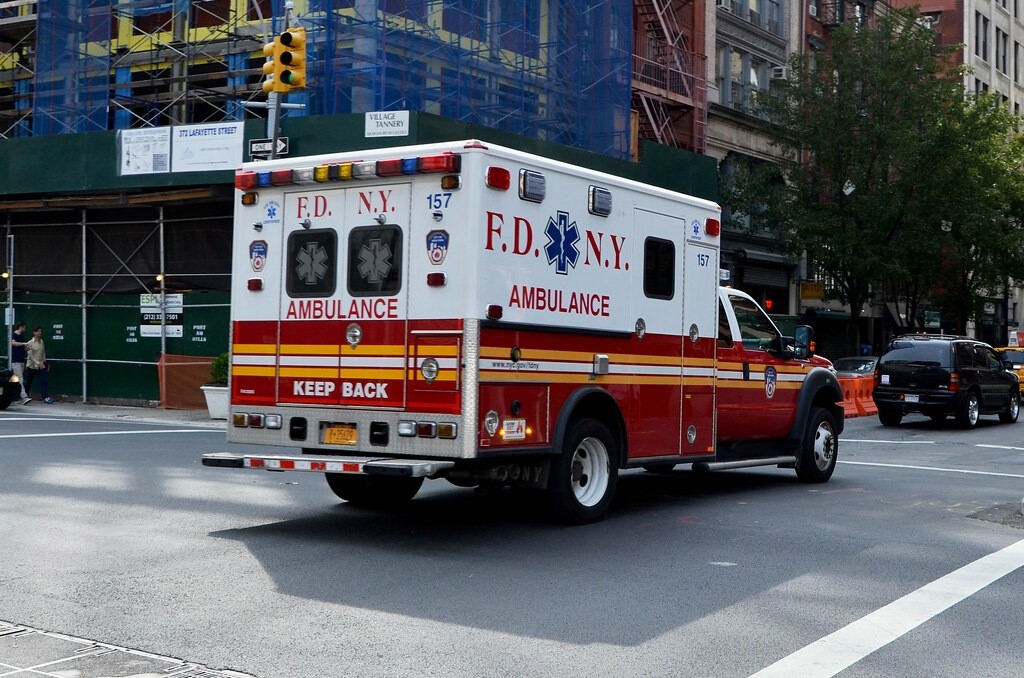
[200,136,849,511]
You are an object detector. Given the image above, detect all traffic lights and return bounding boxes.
[260,27,307,93]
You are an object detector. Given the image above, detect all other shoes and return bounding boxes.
[14,400,20,404]
[43,397,54,404]
[22,397,32,405]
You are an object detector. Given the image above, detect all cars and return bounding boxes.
[990,345,1024,392]
[872,331,1021,428]
[830,357,880,388]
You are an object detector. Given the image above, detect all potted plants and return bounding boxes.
[200,351,229,420]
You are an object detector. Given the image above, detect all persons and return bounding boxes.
[12,322,54,405]
